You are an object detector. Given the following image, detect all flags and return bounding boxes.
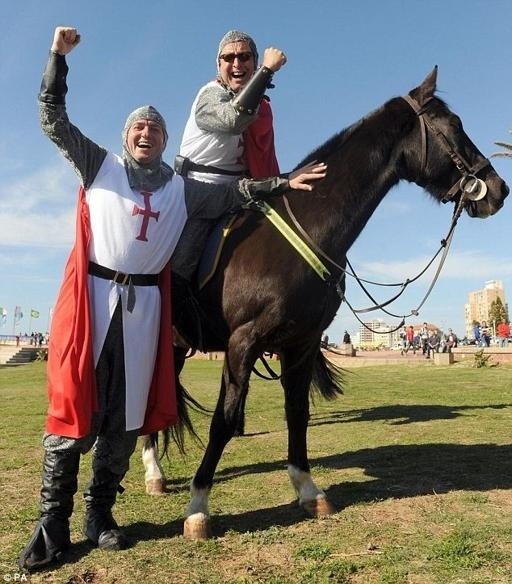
[0,307,6,316]
[31,309,39,318]
[15,307,22,318]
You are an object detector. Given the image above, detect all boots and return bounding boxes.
[20,448,78,569]
[83,466,128,551]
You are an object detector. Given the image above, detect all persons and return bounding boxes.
[20,25,328,571]
[475,318,510,348]
[169,30,287,314]
[343,331,350,344]
[16,332,43,347]
[400,323,457,359]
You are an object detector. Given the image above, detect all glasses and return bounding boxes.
[219,51,252,63]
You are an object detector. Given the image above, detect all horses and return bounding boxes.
[138,59,512,543]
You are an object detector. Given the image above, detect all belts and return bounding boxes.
[87,261,159,287]
[188,163,248,176]
